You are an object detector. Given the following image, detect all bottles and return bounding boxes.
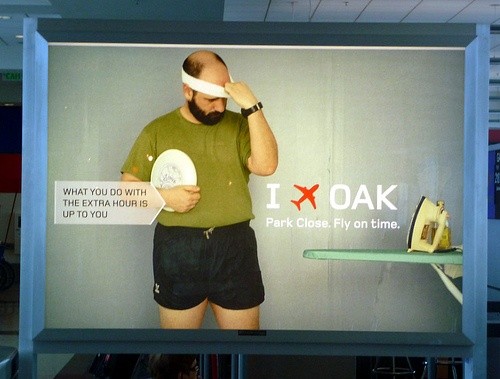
[431,199,452,251]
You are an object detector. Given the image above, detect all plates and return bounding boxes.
[148,148,198,213]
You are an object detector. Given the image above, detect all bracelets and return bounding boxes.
[241,101,263,119]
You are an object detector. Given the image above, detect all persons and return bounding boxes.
[120,47,279,332]
[147,352,200,379]
